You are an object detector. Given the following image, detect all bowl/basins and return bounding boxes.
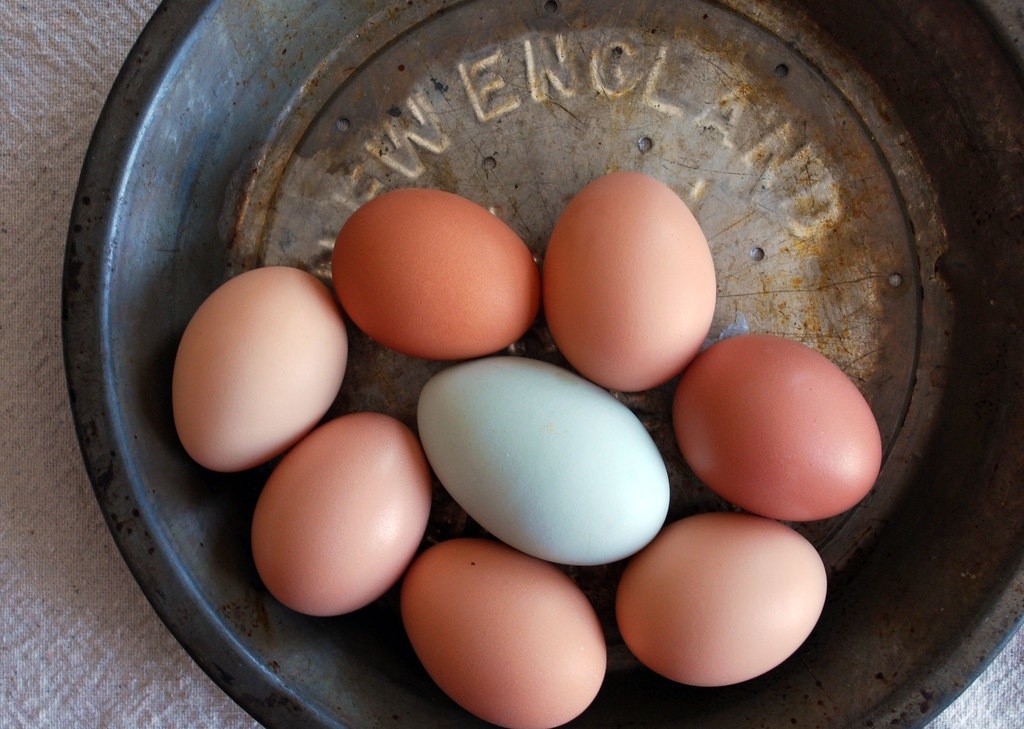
[60,0,1024,729]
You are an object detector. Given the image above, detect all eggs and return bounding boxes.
[170,174,881,729]
